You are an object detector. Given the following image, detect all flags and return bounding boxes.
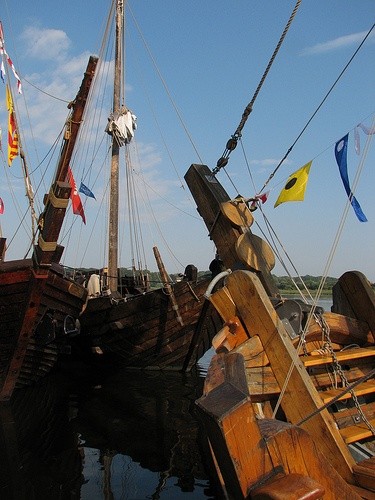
[78,182,97,202]
[274,161,311,207]
[5,85,21,166]
[69,169,86,223]
[5,91,18,165]
[335,134,368,224]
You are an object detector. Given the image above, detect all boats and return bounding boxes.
[1,0,375,500]
